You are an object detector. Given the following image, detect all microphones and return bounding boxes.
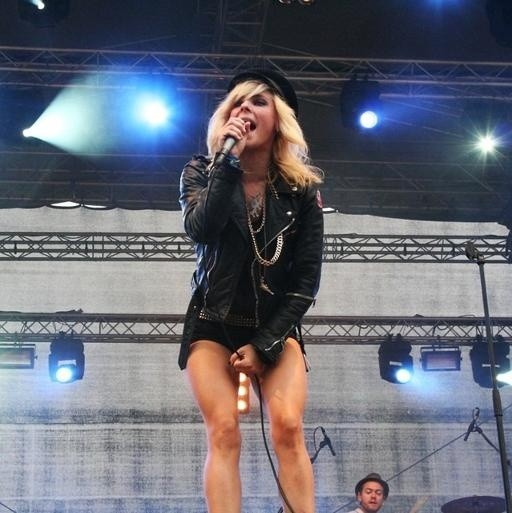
[321,425,338,459]
[213,134,236,168]
[462,413,481,443]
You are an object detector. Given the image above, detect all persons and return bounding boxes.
[177,68,325,513]
[348,473,389,513]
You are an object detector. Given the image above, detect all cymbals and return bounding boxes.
[440,496,505,512]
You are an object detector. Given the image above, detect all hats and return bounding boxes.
[355,472,389,499]
[227,55,299,119]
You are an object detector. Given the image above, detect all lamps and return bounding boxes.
[466,341,509,390]
[378,337,418,388]
[336,81,382,133]
[43,339,86,383]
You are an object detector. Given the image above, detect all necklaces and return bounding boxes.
[237,174,284,267]
